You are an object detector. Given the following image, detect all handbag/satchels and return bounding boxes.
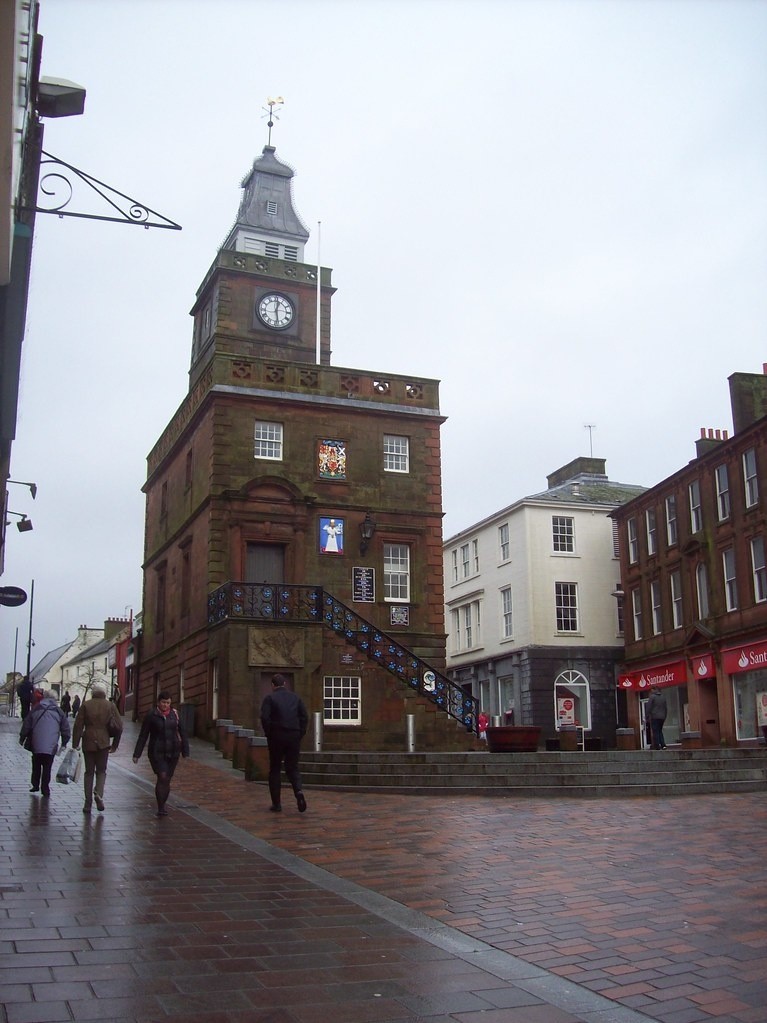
[176,731,183,752]
[109,703,121,736]
[24,729,32,751]
[56,749,83,785]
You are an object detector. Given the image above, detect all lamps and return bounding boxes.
[358,511,378,556]
[6,478,37,531]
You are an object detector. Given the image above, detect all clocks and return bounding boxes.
[256,291,296,331]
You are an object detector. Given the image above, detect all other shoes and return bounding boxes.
[29,787,39,792]
[43,791,49,797]
[663,746,667,749]
[296,792,306,812]
[83,808,91,813]
[94,795,105,810]
[270,805,281,811]
[158,810,168,815]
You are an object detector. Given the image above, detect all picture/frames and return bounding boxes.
[315,514,348,558]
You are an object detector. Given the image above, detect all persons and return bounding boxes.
[132,692,190,814]
[17,675,44,722]
[646,686,667,750]
[113,684,121,715]
[260,673,308,813]
[478,712,488,746]
[60,691,71,718]
[325,519,341,552]
[72,695,80,718]
[72,685,124,813]
[19,690,70,793]
